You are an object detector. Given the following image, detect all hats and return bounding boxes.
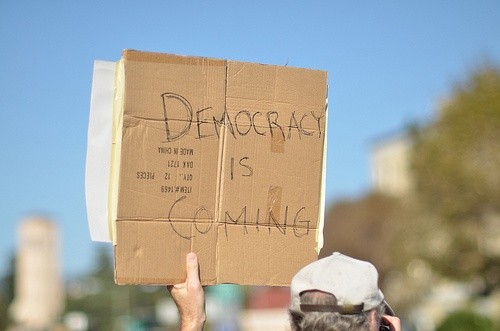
[289,250,395,318]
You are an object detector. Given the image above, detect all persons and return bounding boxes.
[167,249,401,331]
